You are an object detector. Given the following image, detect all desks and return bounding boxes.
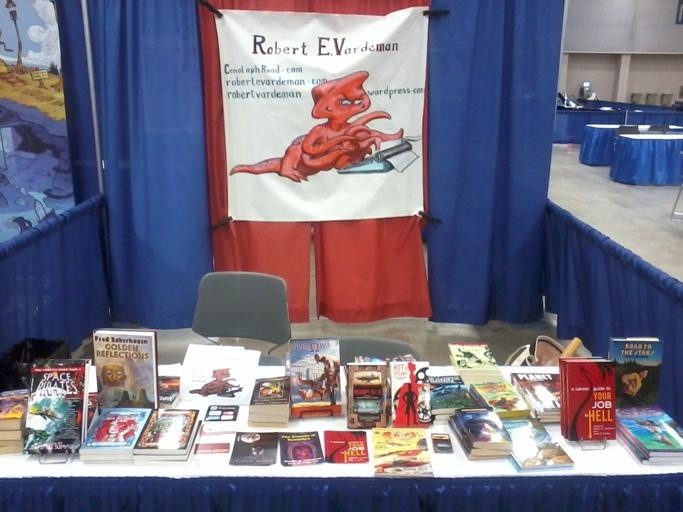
[0,365,680,509]
[552,99,683,187]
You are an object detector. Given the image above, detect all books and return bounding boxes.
[0,330,683,478]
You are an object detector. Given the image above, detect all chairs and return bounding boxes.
[190,270,293,370]
